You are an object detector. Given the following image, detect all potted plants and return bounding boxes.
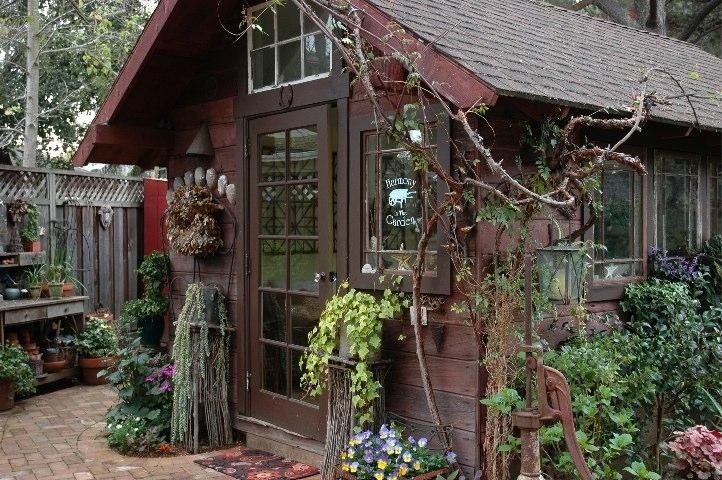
[0,202,170,413]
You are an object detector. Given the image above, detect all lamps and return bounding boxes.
[535,220,587,305]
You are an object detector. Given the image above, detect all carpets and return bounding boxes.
[192,446,320,480]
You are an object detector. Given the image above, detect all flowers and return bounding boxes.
[338,423,458,480]
[104,361,173,454]
